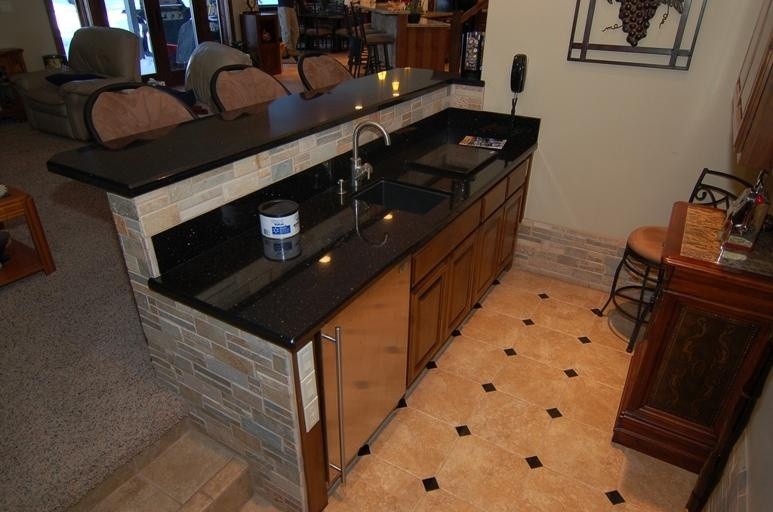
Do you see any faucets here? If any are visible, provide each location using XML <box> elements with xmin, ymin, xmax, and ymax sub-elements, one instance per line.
<box><xmin>350</xmin><ymin>119</ymin><xmax>392</xmax><ymax>184</ymax></box>
<box><xmin>353</xmin><ymin>200</ymin><xmax>390</xmax><ymax>248</ymax></box>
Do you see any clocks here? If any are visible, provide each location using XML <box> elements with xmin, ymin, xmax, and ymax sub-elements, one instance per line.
<box><xmin>247</xmin><ymin>0</ymin><xmax>256</xmax><ymax>8</ymax></box>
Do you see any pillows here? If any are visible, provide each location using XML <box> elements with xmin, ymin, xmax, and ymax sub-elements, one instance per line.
<box><xmin>45</xmin><ymin>73</ymin><xmax>108</xmax><ymax>85</ymax></box>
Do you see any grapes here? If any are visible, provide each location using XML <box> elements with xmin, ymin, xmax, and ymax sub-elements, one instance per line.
<box><xmin>619</xmin><ymin>0</ymin><xmax>660</xmax><ymax>47</ymax></box>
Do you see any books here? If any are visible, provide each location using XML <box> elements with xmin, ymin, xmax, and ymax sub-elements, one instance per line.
<box><xmin>460</xmin><ymin>134</ymin><xmax>508</xmax><ymax>150</ymax></box>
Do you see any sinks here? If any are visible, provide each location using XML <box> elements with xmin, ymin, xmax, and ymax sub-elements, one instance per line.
<box><xmin>351</xmin><ymin>179</ymin><xmax>451</xmax><ymax>216</ymax></box>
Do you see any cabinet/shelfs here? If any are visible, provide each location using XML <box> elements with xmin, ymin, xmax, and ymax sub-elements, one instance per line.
<box><xmin>314</xmin><ymin>256</ymin><xmax>410</xmax><ymax>487</ymax></box>
<box><xmin>473</xmin><ymin>157</ymin><xmax>529</xmax><ymax>307</ymax></box>
<box><xmin>409</xmin><ymin>201</ymin><xmax>483</xmax><ymax>391</ymax></box>
<box><xmin>241</xmin><ymin>11</ymin><xmax>282</xmax><ymax>75</ymax></box>
<box><xmin>611</xmin><ymin>250</ymin><xmax>773</xmax><ymax>477</ymax></box>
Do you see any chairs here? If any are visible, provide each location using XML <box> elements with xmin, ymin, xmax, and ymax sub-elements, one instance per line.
<box><xmin>600</xmin><ymin>167</ymin><xmax>755</xmax><ymax>354</ymax></box>
<box><xmin>296</xmin><ymin>0</ymin><xmax>394</xmax><ymax>77</ymax></box>
<box><xmin>210</xmin><ymin>64</ymin><xmax>293</xmax><ymax>110</ymax></box>
<box><xmin>184</xmin><ymin>42</ymin><xmax>253</xmax><ymax>119</ymax></box>
<box><xmin>10</xmin><ymin>27</ymin><xmax>142</xmax><ymax>141</ymax></box>
<box><xmin>297</xmin><ymin>53</ymin><xmax>355</xmax><ymax>90</ymax></box>
<box><xmin>83</xmin><ymin>82</ymin><xmax>200</xmax><ymax>144</ymax></box>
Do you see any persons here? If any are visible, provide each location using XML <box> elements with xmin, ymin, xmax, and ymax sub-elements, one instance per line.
<box><xmin>275</xmin><ymin>0</ymin><xmax>302</xmax><ymax>52</ymax></box>
